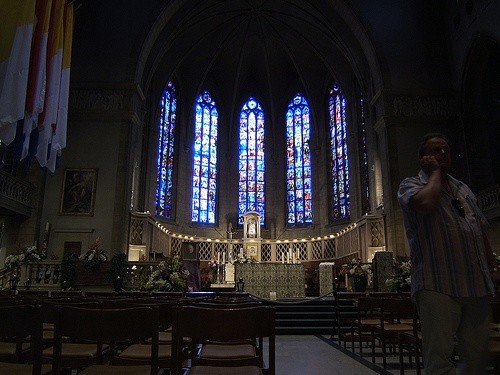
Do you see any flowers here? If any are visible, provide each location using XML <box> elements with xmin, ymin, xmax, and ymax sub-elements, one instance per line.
<box><xmin>340</xmin><ymin>258</ymin><xmax>372</xmax><ymax>284</ymax></box>
<box><xmin>385</xmin><ymin>256</ymin><xmax>414</xmax><ymax>291</ymax></box>
<box><xmin>5</xmin><ymin>241</ymin><xmax>40</xmax><ymax>267</ymax></box>
<box><xmin>79</xmin><ymin>238</ymin><xmax>108</xmax><ymax>270</ymax></box>
<box><xmin>145</xmin><ymin>260</ymin><xmax>191</xmax><ymax>291</ymax></box>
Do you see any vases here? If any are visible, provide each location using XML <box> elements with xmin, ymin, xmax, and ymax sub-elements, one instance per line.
<box><xmin>15</xmin><ymin>262</ymin><xmax>32</xmax><ymax>286</ymax></box>
<box><xmin>350</xmin><ymin>276</ymin><xmax>366</xmax><ymax>291</ymax></box>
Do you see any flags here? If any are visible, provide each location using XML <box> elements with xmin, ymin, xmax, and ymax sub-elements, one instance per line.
<box><xmin>0</xmin><ymin>0</ymin><xmax>74</xmax><ymax>175</ymax></box>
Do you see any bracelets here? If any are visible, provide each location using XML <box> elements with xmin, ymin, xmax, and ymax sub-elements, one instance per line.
<box><xmin>489</xmin><ymin>267</ymin><xmax>499</xmax><ymax>272</ymax></box>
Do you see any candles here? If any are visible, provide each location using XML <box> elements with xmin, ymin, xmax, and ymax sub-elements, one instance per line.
<box><xmin>46</xmin><ymin>222</ymin><xmax>49</xmax><ymax>232</ymax></box>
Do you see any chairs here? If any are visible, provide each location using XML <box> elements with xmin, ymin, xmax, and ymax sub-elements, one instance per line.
<box><xmin>331</xmin><ymin>292</ymin><xmax>500</xmax><ymax>375</ymax></box>
<box><xmin>0</xmin><ymin>290</ymin><xmax>278</xmax><ymax>375</ymax></box>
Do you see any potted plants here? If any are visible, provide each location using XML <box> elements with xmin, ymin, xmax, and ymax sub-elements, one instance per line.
<box><xmin>110</xmin><ymin>252</ymin><xmax>127</xmax><ymax>292</ymax></box>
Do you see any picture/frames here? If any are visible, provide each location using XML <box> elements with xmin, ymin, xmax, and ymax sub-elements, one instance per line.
<box><xmin>59</xmin><ymin>168</ymin><xmax>98</xmax><ymax>216</ymax></box>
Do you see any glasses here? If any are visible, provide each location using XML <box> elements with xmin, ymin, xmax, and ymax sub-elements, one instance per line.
<box><xmin>451</xmin><ymin>197</ymin><xmax>466</xmax><ymax>218</ymax></box>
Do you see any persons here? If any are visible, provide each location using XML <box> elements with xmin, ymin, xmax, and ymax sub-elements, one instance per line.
<box><xmin>397</xmin><ymin>132</ymin><xmax>500</xmax><ymax>375</ymax></box>
